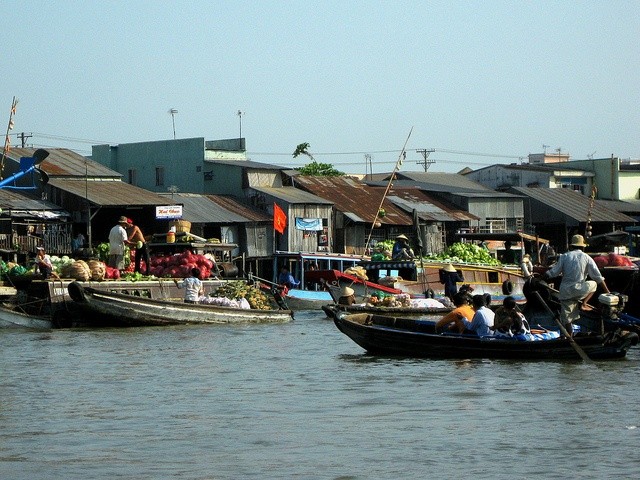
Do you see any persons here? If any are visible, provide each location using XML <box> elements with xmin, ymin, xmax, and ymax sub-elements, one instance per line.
<box><xmin>35</xmin><ymin>247</ymin><xmax>53</xmax><ymax>280</ymax></box>
<box><xmin>107</xmin><ymin>216</ymin><xmax>137</xmax><ymax>272</ymax></box>
<box><xmin>489</xmin><ymin>296</ymin><xmax>530</xmax><ymax>338</ymax></box>
<box><xmin>173</xmin><ymin>268</ymin><xmax>203</xmax><ymax>305</ymax></box>
<box><xmin>339</xmin><ymin>286</ymin><xmax>356</xmax><ymax>306</ymax></box>
<box><xmin>72</xmin><ymin>237</ymin><xmax>77</xmax><ymax>253</ymax></box>
<box><xmin>435</xmin><ymin>293</ymin><xmax>476</xmax><ymax>333</ymax></box>
<box><xmin>501</xmin><ymin>240</ymin><xmax>515</xmax><ymax>263</ymax></box>
<box><xmin>440</xmin><ymin>264</ymin><xmax>464</xmax><ymax>300</ymax></box>
<box><xmin>305</xmin><ymin>264</ymin><xmax>321</xmax><ymax>290</ymax></box>
<box><xmin>392</xmin><ymin>233</ymin><xmax>415</xmax><ymax>262</ymax></box>
<box><xmin>533</xmin><ymin>234</ymin><xmax>611</xmax><ymax>337</ymax></box>
<box><xmin>456</xmin><ymin>295</ymin><xmax>496</xmax><ymax>340</ymax></box>
<box><xmin>125</xmin><ymin>219</ymin><xmax>150</xmax><ymax>276</ymax></box>
<box><xmin>77</xmin><ymin>232</ymin><xmax>84</xmax><ymax>248</ymax></box>
<box><xmin>279</xmin><ymin>266</ymin><xmax>300</xmax><ymax>290</ymax></box>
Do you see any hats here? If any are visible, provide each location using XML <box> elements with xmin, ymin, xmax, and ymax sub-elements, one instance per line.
<box><xmin>118</xmin><ymin>216</ymin><xmax>127</xmax><ymax>223</ymax></box>
<box><xmin>443</xmin><ymin>264</ymin><xmax>457</xmax><ymax>273</ymax></box>
<box><xmin>340</xmin><ymin>287</ymin><xmax>354</xmax><ymax>296</ymax></box>
<box><xmin>37</xmin><ymin>247</ymin><xmax>44</xmax><ymax>251</ymax></box>
<box><xmin>570</xmin><ymin>235</ymin><xmax>586</xmax><ymax>247</ymax></box>
<box><xmin>396</xmin><ymin>234</ymin><xmax>408</xmax><ymax>240</ymax></box>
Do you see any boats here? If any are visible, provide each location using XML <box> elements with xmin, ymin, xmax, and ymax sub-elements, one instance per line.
<box><xmin>334</xmin><ymin>292</ymin><xmax>639</xmax><ymax>360</ymax></box>
<box><xmin>271</xmin><ymin>250</ymin><xmax>371</xmax><ymax>309</ymax></box>
<box><xmin>67</xmin><ymin>281</ymin><xmax>295</xmax><ymax>326</ymax></box>
<box><xmin>322</xmin><ymin>303</ymin><xmax>456</xmax><ymax>318</ymax></box>
<box><xmin>0</xmin><ymin>243</ymin><xmax>249</xmax><ymax>329</ymax></box>
<box><xmin>541</xmin><ymin>253</ymin><xmax>640</xmax><ymax>320</ymax></box>
<box><xmin>306</xmin><ymin>232</ymin><xmax>549</xmax><ymax>304</ymax></box>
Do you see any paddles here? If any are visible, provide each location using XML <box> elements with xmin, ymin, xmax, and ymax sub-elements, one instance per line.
<box><xmin>534</xmin><ymin>289</ymin><xmax>593</xmax><ymax>364</ymax></box>
